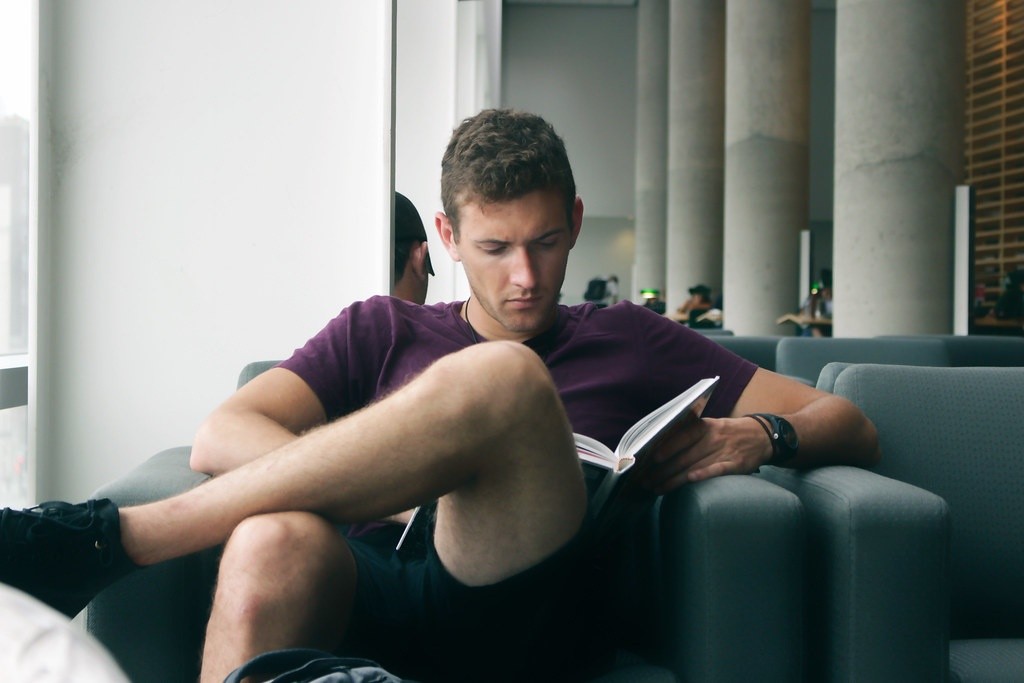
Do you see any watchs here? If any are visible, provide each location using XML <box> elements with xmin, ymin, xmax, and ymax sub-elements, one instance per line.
<box><xmin>755</xmin><ymin>413</ymin><xmax>800</xmax><ymax>461</ymax></box>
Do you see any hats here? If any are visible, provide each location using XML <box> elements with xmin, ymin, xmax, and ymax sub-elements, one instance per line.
<box><xmin>688</xmin><ymin>284</ymin><xmax>712</xmax><ymax>303</ymax></box>
<box><xmin>395</xmin><ymin>190</ymin><xmax>436</xmax><ymax>277</ymax></box>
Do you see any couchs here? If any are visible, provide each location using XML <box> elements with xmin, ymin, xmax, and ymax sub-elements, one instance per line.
<box><xmin>749</xmin><ymin>361</ymin><xmax>1024</xmax><ymax>683</ymax></box>
<box><xmin>688</xmin><ymin>328</ymin><xmax>1024</xmax><ymax>387</ymax></box>
<box><xmin>86</xmin><ymin>361</ymin><xmax>811</xmax><ymax>683</ymax></box>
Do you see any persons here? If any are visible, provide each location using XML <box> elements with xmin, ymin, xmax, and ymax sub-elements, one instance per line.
<box><xmin>803</xmin><ymin>270</ymin><xmax>831</xmax><ymax>336</ymax></box>
<box><xmin>643</xmin><ymin>293</ymin><xmax>665</xmax><ymax>315</ymax></box>
<box><xmin>1</xmin><ymin>107</ymin><xmax>880</xmax><ymax>683</ymax></box>
<box><xmin>995</xmin><ymin>269</ymin><xmax>1024</xmax><ymax>322</ymax></box>
<box><xmin>392</xmin><ymin>191</ymin><xmax>436</xmax><ymax>304</ymax></box>
<box><xmin>583</xmin><ymin>275</ymin><xmax>618</xmax><ymax>310</ymax></box>
<box><xmin>675</xmin><ymin>284</ymin><xmax>712</xmax><ymax>328</ymax></box>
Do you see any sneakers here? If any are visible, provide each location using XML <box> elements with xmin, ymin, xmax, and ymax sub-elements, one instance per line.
<box><xmin>0</xmin><ymin>497</ymin><xmax>133</xmax><ymax>618</ymax></box>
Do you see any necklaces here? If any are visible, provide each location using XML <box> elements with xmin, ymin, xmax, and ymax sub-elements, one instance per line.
<box><xmin>466</xmin><ymin>294</ymin><xmax>560</xmax><ymax>364</ymax></box>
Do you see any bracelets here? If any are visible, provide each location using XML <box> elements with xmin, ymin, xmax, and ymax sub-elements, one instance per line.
<box><xmin>742</xmin><ymin>414</ymin><xmax>776</xmax><ymax>463</ymax></box>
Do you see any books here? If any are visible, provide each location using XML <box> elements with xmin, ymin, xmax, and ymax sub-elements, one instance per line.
<box><xmin>394</xmin><ymin>376</ymin><xmax>721</xmax><ymax>552</ymax></box>
